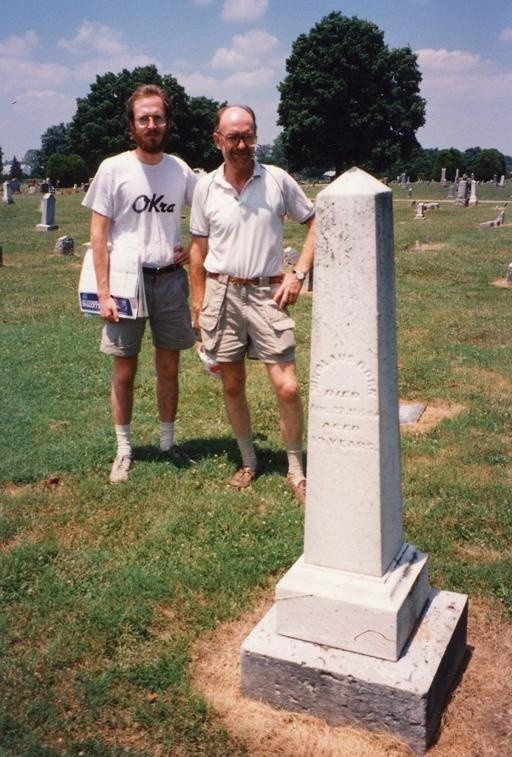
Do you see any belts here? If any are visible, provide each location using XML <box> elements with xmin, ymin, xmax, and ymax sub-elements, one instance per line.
<box><xmin>143</xmin><ymin>264</ymin><xmax>182</xmax><ymax>273</ymax></box>
<box><xmin>204</xmin><ymin>271</ymin><xmax>282</xmax><ymax>285</ymax></box>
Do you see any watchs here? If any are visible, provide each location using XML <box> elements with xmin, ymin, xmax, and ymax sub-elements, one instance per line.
<box><xmin>292</xmin><ymin>268</ymin><xmax>305</xmax><ymax>282</ymax></box>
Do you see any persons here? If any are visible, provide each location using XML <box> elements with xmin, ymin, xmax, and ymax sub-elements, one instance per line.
<box><xmin>188</xmin><ymin>105</ymin><xmax>315</xmax><ymax>503</ymax></box>
<box><xmin>82</xmin><ymin>84</ymin><xmax>200</xmax><ymax>485</ymax></box>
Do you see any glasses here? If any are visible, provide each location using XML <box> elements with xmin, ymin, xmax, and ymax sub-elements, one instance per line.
<box><xmin>135</xmin><ymin>115</ymin><xmax>166</xmax><ymax>127</ymax></box>
<box><xmin>225</xmin><ymin>134</ymin><xmax>257</xmax><ymax>146</ymax></box>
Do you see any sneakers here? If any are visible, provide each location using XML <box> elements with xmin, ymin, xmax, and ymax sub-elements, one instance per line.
<box><xmin>157</xmin><ymin>443</ymin><xmax>196</xmax><ymax>466</ymax></box>
<box><xmin>109</xmin><ymin>449</ymin><xmax>134</xmax><ymax>485</ymax></box>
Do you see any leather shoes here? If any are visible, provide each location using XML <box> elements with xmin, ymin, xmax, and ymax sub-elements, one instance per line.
<box><xmin>286</xmin><ymin>468</ymin><xmax>306</xmax><ymax>506</ymax></box>
<box><xmin>230</xmin><ymin>464</ymin><xmax>263</xmax><ymax>487</ymax></box>
